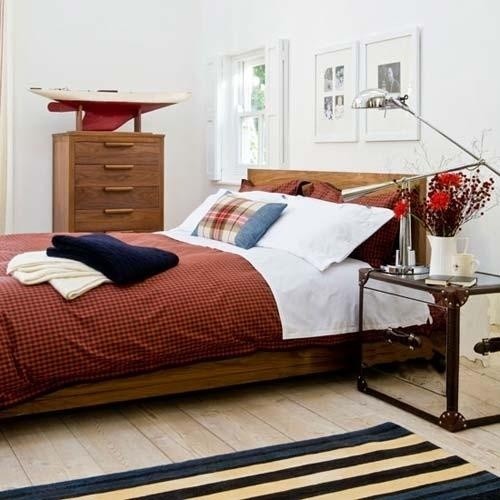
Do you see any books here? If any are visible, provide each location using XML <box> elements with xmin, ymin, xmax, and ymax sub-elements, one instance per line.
<box><xmin>425</xmin><ymin>275</ymin><xmax>477</xmax><ymax>287</ymax></box>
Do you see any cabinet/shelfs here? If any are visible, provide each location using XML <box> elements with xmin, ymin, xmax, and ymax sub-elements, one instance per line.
<box><xmin>51</xmin><ymin>132</ymin><xmax>166</xmax><ymax>235</ymax></box>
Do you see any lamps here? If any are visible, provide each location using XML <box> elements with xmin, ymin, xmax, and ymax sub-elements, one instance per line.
<box><xmin>341</xmin><ymin>89</ymin><xmax>500</xmax><ymax>276</ymax></box>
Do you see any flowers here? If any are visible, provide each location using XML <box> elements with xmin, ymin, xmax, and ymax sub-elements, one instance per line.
<box><xmin>390</xmin><ymin>168</ymin><xmax>498</xmax><ymax>237</ymax></box>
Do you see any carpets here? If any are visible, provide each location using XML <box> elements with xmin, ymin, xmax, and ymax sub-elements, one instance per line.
<box><xmin>0</xmin><ymin>420</ymin><xmax>500</xmax><ymax>499</ymax></box>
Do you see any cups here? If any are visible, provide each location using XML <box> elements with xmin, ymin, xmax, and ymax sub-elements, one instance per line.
<box><xmin>450</xmin><ymin>253</ymin><xmax>479</xmax><ymax>277</ymax></box>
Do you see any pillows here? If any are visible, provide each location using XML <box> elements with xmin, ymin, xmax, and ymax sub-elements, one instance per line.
<box><xmin>176</xmin><ymin>177</ymin><xmax>420</xmax><ymax>273</ymax></box>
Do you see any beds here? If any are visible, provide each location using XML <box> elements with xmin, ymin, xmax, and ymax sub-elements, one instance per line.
<box><xmin>0</xmin><ymin>168</ymin><xmax>429</xmax><ymax>417</ymax></box>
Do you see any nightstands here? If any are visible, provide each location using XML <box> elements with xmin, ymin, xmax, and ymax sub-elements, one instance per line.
<box><xmin>355</xmin><ymin>266</ymin><xmax>500</xmax><ymax>432</ymax></box>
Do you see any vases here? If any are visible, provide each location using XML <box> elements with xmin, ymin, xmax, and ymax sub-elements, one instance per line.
<box><xmin>426</xmin><ymin>234</ymin><xmax>469</xmax><ymax>280</ymax></box>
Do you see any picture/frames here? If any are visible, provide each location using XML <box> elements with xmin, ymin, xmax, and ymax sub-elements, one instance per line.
<box><xmin>312</xmin><ymin>27</ymin><xmax>422</xmax><ymax>144</ymax></box>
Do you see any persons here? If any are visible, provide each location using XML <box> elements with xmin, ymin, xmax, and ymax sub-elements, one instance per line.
<box><xmin>324</xmin><ymin>65</ymin><xmax>344</xmax><ymax>119</ymax></box>
<box><xmin>385</xmin><ymin>67</ymin><xmax>400</xmax><ymax>92</ymax></box>
